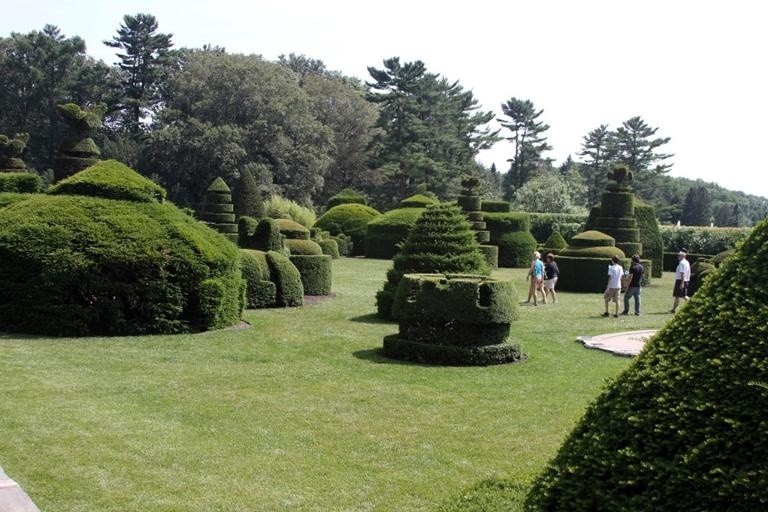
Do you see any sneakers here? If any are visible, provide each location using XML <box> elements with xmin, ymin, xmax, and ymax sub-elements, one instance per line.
<box><xmin>521</xmin><ymin>299</ymin><xmax>559</xmax><ymax>306</ymax></box>
<box><xmin>667</xmin><ymin>309</ymin><xmax>676</xmax><ymax>313</ymax></box>
<box><xmin>601</xmin><ymin>309</ymin><xmax>641</xmax><ymax>318</ymax></box>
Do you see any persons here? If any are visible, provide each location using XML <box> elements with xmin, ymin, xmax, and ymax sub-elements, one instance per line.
<box><xmin>525</xmin><ymin>250</ymin><xmax>544</xmax><ymax>306</ymax></box>
<box><xmin>529</xmin><ymin>259</ymin><xmax>548</xmax><ymax>306</ymax></box>
<box><xmin>670</xmin><ymin>250</ymin><xmax>691</xmax><ymax>314</ymax></box>
<box><xmin>542</xmin><ymin>253</ymin><xmax>561</xmax><ymax>305</ymax></box>
<box><xmin>619</xmin><ymin>254</ymin><xmax>645</xmax><ymax>316</ymax></box>
<box><xmin>601</xmin><ymin>254</ymin><xmax>625</xmax><ymax>318</ymax></box>
